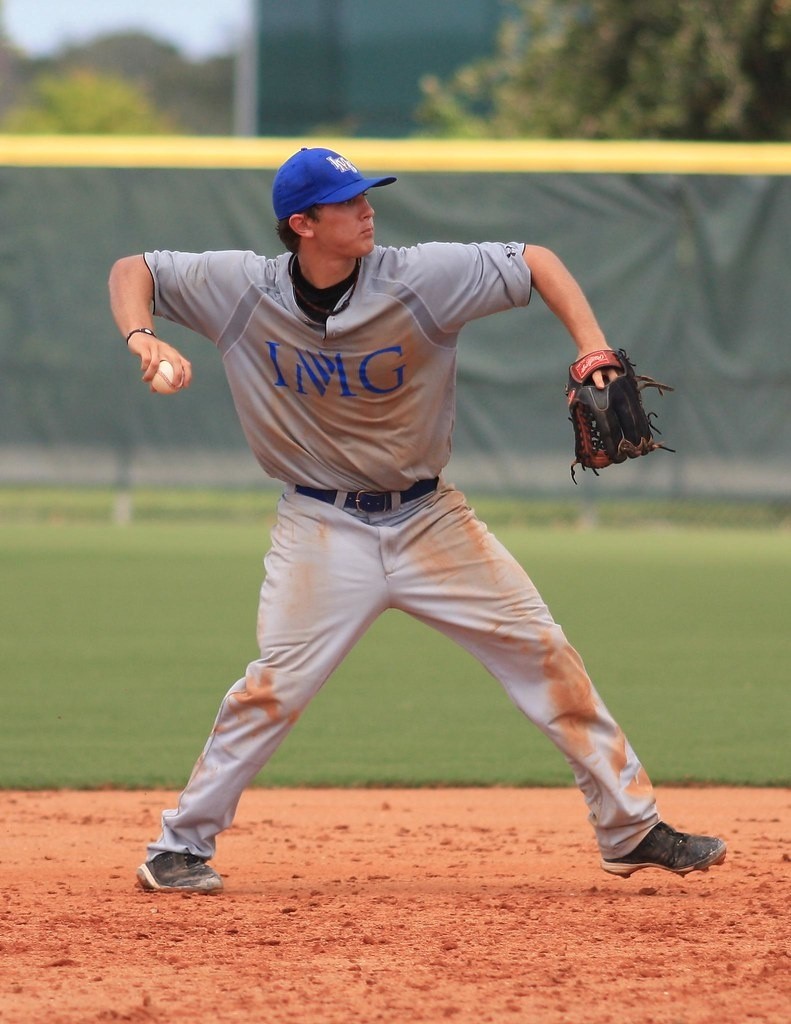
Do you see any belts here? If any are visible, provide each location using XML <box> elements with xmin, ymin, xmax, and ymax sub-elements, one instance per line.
<box><xmin>295</xmin><ymin>476</ymin><xmax>439</xmax><ymax>512</ymax></box>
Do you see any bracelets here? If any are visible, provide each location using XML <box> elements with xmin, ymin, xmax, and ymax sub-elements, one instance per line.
<box><xmin>126</xmin><ymin>328</ymin><xmax>156</xmax><ymax>345</ymax></box>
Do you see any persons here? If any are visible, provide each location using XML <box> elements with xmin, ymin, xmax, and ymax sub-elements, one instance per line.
<box><xmin>108</xmin><ymin>147</ymin><xmax>728</xmax><ymax>894</ymax></box>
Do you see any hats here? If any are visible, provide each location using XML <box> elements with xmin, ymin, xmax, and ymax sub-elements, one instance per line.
<box><xmin>271</xmin><ymin>147</ymin><xmax>397</xmax><ymax>221</ymax></box>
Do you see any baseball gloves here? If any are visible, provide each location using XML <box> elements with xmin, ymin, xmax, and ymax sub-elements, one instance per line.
<box><xmin>560</xmin><ymin>346</ymin><xmax>676</xmax><ymax>486</ymax></box>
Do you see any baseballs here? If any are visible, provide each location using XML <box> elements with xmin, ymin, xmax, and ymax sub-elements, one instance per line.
<box><xmin>150</xmin><ymin>360</ymin><xmax>186</xmax><ymax>396</ymax></box>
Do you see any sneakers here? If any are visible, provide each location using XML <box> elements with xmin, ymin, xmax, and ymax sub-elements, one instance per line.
<box><xmin>136</xmin><ymin>847</ymin><xmax>224</xmax><ymax>895</ymax></box>
<box><xmin>600</xmin><ymin>820</ymin><xmax>727</xmax><ymax>876</ymax></box>
